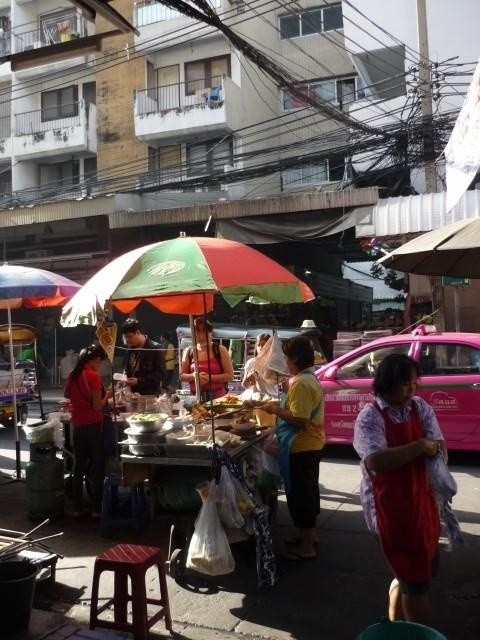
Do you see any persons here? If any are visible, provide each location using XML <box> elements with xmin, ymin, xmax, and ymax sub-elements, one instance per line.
<box><xmin>260</xmin><ymin>335</ymin><xmax>327</xmax><ymax>562</ymax></box>
<box><xmin>120</xmin><ymin>317</ymin><xmax>166</xmax><ymax>396</ymax></box>
<box><xmin>178</xmin><ymin>316</ymin><xmax>235</xmax><ymax>401</ymax></box>
<box><xmin>351</xmin><ymin>352</ymin><xmax>459</xmax><ymax>622</ymax></box>
<box><xmin>159</xmin><ymin>330</ymin><xmax>175</xmax><ymax>380</ymax></box>
<box><xmin>63</xmin><ymin>343</ymin><xmax>117</xmax><ymax>516</ymax></box>
<box><xmin>239</xmin><ymin>333</ymin><xmax>295</xmax><ymax>427</ymax></box>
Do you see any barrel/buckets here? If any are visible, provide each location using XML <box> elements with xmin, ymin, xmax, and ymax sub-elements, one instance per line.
<box><xmin>0</xmin><ymin>558</ymin><xmax>40</xmax><ymax>634</ymax></box>
<box><xmin>332</xmin><ymin>328</ymin><xmax>392</xmax><ymax>357</ymax></box>
<box><xmin>358</xmin><ymin>617</ymin><xmax>446</xmax><ymax>640</ymax></box>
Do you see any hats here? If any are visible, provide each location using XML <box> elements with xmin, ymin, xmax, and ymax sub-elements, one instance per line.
<box><xmin>421</xmin><ymin>316</ymin><xmax>433</xmax><ymax>324</ymax></box>
<box><xmin>300</xmin><ymin>320</ymin><xmax>317</xmax><ymax>328</ymax></box>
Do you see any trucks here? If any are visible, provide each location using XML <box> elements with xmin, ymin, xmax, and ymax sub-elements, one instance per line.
<box><xmin>0</xmin><ymin>359</ymin><xmax>37</xmax><ymax>427</ymax></box>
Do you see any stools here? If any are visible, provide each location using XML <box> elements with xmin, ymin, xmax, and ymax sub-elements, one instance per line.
<box><xmin>89</xmin><ymin>542</ymin><xmax>173</xmax><ymax>638</ymax></box>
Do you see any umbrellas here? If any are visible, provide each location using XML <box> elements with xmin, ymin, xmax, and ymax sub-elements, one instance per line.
<box><xmin>375</xmin><ymin>217</ymin><xmax>480</xmax><ymax>279</ymax></box>
<box><xmin>60</xmin><ymin>230</ymin><xmax>316</xmax><ymax>403</ymax></box>
<box><xmin>0</xmin><ymin>260</ymin><xmax>84</xmax><ymax>478</ymax></box>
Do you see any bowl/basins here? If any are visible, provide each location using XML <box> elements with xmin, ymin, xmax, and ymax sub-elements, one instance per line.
<box><xmin>233</xmin><ymin>420</ymin><xmax>257</xmax><ymax>432</ymax></box>
<box><xmin>124</xmin><ymin>414</ymin><xmax>170</xmax><ymax>456</ymax></box>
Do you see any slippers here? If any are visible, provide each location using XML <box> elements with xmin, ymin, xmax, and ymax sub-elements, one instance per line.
<box><xmin>279</xmin><ymin>536</ymin><xmax>320</xmax><ymax>562</ymax></box>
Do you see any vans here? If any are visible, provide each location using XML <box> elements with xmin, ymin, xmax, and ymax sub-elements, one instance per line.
<box><xmin>176</xmin><ymin>320</ymin><xmax>329</xmax><ymax>413</ymax></box>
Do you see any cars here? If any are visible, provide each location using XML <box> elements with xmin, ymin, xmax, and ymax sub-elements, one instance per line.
<box><xmin>314</xmin><ymin>324</ymin><xmax>480</xmax><ymax>457</ymax></box>
